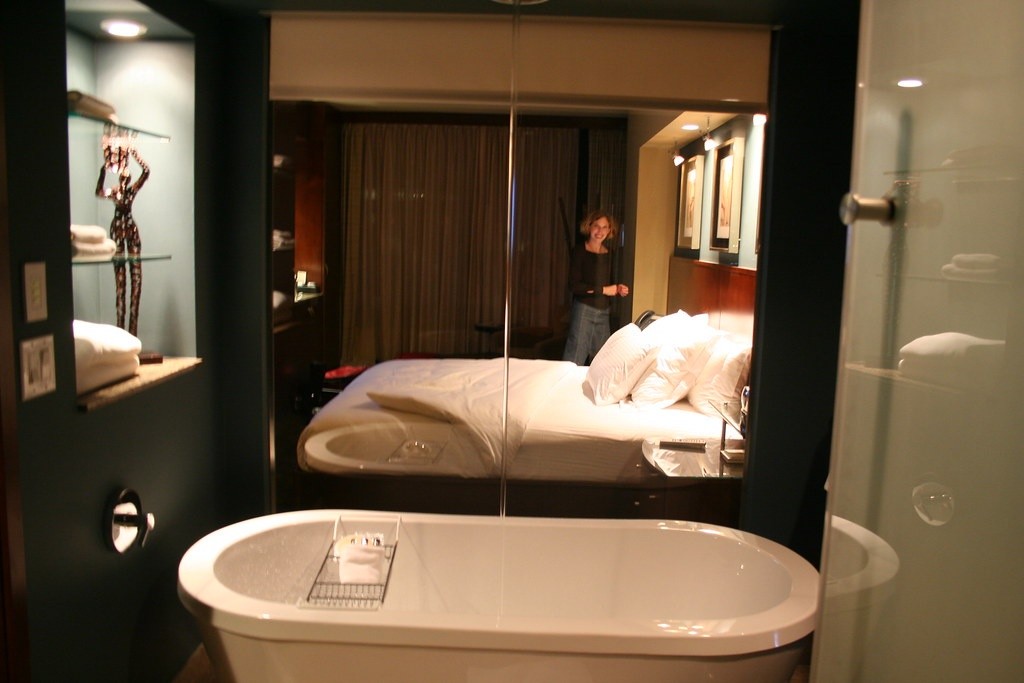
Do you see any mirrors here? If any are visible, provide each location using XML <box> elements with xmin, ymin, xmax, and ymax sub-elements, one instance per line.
<box><xmin>258</xmin><ymin>11</ymin><xmax>774</xmax><ymax>527</ymax></box>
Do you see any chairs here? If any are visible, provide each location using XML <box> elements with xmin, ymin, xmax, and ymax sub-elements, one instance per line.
<box><xmin>491</xmin><ymin>325</ymin><xmax>553</xmax><ymax>360</ymax></box>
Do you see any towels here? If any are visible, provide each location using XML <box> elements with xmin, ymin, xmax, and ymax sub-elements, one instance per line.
<box><xmin>75</xmin><ymin>317</ymin><xmax>144</xmax><ymax>392</ymax></box>
<box><xmin>72</xmin><ymin>224</ymin><xmax>118</xmax><ymax>264</ymax></box>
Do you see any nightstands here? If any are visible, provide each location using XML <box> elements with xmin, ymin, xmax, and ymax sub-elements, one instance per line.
<box><xmin>636</xmin><ymin>437</ymin><xmax>744</xmax><ymax>530</ymax></box>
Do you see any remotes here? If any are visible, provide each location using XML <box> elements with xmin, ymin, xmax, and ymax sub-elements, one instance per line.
<box><xmin>659</xmin><ymin>437</ymin><xmax>706</xmax><ymax>447</ymax></box>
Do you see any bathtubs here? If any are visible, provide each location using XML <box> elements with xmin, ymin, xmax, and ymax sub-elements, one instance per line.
<box><xmin>179</xmin><ymin>509</ymin><xmax>820</xmax><ymax>683</ymax></box>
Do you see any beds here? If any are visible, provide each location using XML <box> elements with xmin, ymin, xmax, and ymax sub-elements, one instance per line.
<box><xmin>299</xmin><ymin>356</ymin><xmax>747</xmax><ymax>490</ymax></box>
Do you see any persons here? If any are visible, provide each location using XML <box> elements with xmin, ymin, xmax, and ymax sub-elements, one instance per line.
<box><xmin>95</xmin><ymin>146</ymin><xmax>150</xmax><ymax>336</ymax></box>
<box><xmin>564</xmin><ymin>209</ymin><xmax>628</xmax><ymax>366</ymax></box>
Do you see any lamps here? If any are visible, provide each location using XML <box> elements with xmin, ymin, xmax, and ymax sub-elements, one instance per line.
<box><xmin>669</xmin><ymin>140</ymin><xmax>684</xmax><ymax>167</ymax></box>
<box><xmin>698</xmin><ymin>125</ymin><xmax>718</xmax><ymax>152</ymax></box>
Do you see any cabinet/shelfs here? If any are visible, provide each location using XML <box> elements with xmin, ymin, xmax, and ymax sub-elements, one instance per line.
<box><xmin>270</xmin><ymin>140</ymin><xmax>327</xmax><ymax>334</ymax></box>
<box><xmin>62</xmin><ymin>0</ymin><xmax>203</xmax><ymax>415</ymax></box>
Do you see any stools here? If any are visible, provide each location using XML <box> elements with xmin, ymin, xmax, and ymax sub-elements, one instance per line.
<box><xmin>322</xmin><ymin>365</ymin><xmax>367</xmax><ymax>405</ymax></box>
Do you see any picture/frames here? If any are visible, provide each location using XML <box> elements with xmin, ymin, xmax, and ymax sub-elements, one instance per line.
<box><xmin>673</xmin><ymin>155</ymin><xmax>705</xmax><ymax>260</ymax></box>
<box><xmin>709</xmin><ymin>137</ymin><xmax>744</xmax><ymax>254</ymax></box>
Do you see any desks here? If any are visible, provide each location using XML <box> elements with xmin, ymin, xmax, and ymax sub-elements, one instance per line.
<box><xmin>476</xmin><ymin>320</ymin><xmax>505</xmax><ymax>357</ymax></box>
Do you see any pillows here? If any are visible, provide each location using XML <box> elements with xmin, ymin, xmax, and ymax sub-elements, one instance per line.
<box><xmin>586</xmin><ymin>308</ymin><xmax>752</xmax><ymax>418</ymax></box>
